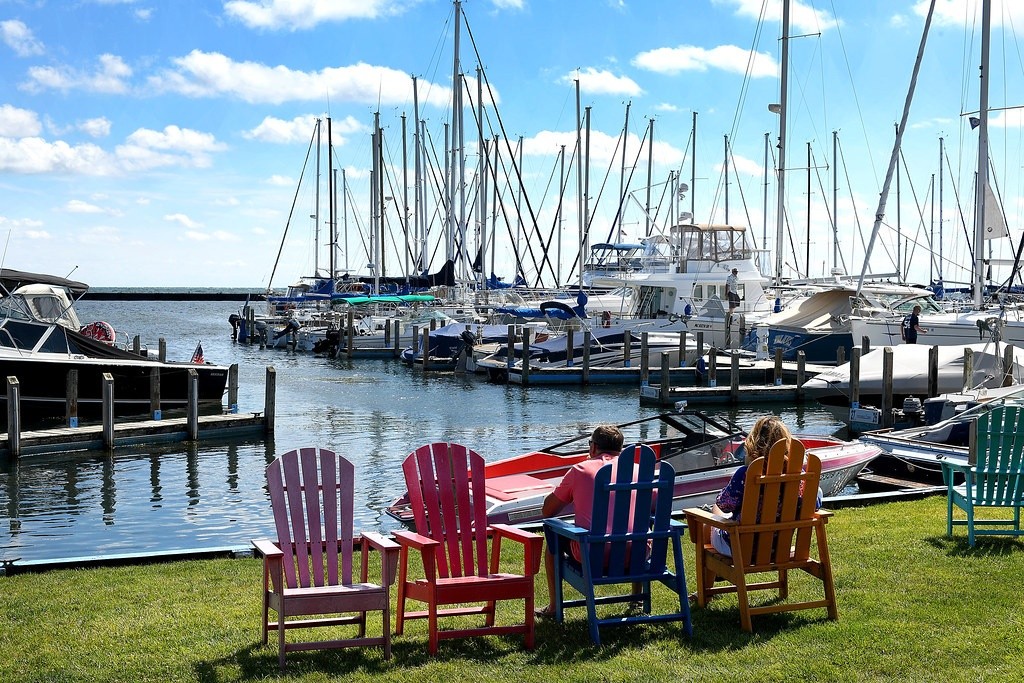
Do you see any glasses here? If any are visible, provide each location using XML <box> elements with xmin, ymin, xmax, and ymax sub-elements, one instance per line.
<box><xmin>588</xmin><ymin>440</ymin><xmax>592</xmax><ymax>446</ymax></box>
<box><xmin>918</xmin><ymin>309</ymin><xmax>921</xmax><ymax>312</ymax></box>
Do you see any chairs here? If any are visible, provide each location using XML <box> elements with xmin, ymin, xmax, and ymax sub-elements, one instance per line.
<box><xmin>542</xmin><ymin>444</ymin><xmax>693</xmax><ymax>646</ymax></box>
<box><xmin>940</xmin><ymin>405</ymin><xmax>1024</xmax><ymax>547</ymax></box>
<box><xmin>389</xmin><ymin>442</ymin><xmax>545</xmax><ymax>658</ymax></box>
<box><xmin>251</xmin><ymin>448</ymin><xmax>402</xmax><ymax>671</ymax></box>
<box><xmin>680</xmin><ymin>437</ymin><xmax>839</xmax><ymax>633</ymax></box>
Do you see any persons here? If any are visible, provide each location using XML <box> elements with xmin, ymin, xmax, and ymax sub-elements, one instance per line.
<box><xmin>688</xmin><ymin>415</ymin><xmax>822</xmax><ymax>602</ymax></box>
<box><xmin>901</xmin><ymin>306</ymin><xmax>927</xmax><ymax>344</ymax></box>
<box><xmin>725</xmin><ymin>268</ymin><xmax>740</xmax><ymax>313</ymax></box>
<box><xmin>534</xmin><ymin>425</ymin><xmax>658</xmax><ymax>617</ymax></box>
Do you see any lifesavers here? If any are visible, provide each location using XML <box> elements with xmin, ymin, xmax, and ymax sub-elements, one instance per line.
<box><xmin>602</xmin><ymin>311</ymin><xmax>611</xmax><ymax>328</ymax></box>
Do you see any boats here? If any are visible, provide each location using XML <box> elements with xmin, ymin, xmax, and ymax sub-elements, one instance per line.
<box><xmin>383</xmin><ymin>409</ymin><xmax>883</xmax><ymax>537</ymax></box>
<box><xmin>0</xmin><ymin>227</ymin><xmax>228</xmax><ymax>418</ymax></box>
<box><xmin>858</xmin><ymin>378</ymin><xmax>1024</xmax><ymax>486</ymax></box>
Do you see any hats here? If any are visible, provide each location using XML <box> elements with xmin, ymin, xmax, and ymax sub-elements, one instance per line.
<box><xmin>732</xmin><ymin>268</ymin><xmax>739</xmax><ymax>272</ymax></box>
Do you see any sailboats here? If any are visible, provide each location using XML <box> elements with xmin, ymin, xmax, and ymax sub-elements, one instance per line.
<box><xmin>231</xmin><ymin>0</ymin><xmax>1024</xmax><ymax>385</ymax></box>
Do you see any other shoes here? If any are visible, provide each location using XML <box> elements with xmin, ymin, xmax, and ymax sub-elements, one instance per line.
<box><xmin>534</xmin><ymin>605</ymin><xmax>565</xmax><ymax>623</ymax></box>
<box><xmin>631</xmin><ymin>601</ymin><xmax>643</xmax><ymax>612</ymax></box>
<box><xmin>689</xmin><ymin>592</ymin><xmax>714</xmax><ymax>605</ymax></box>
